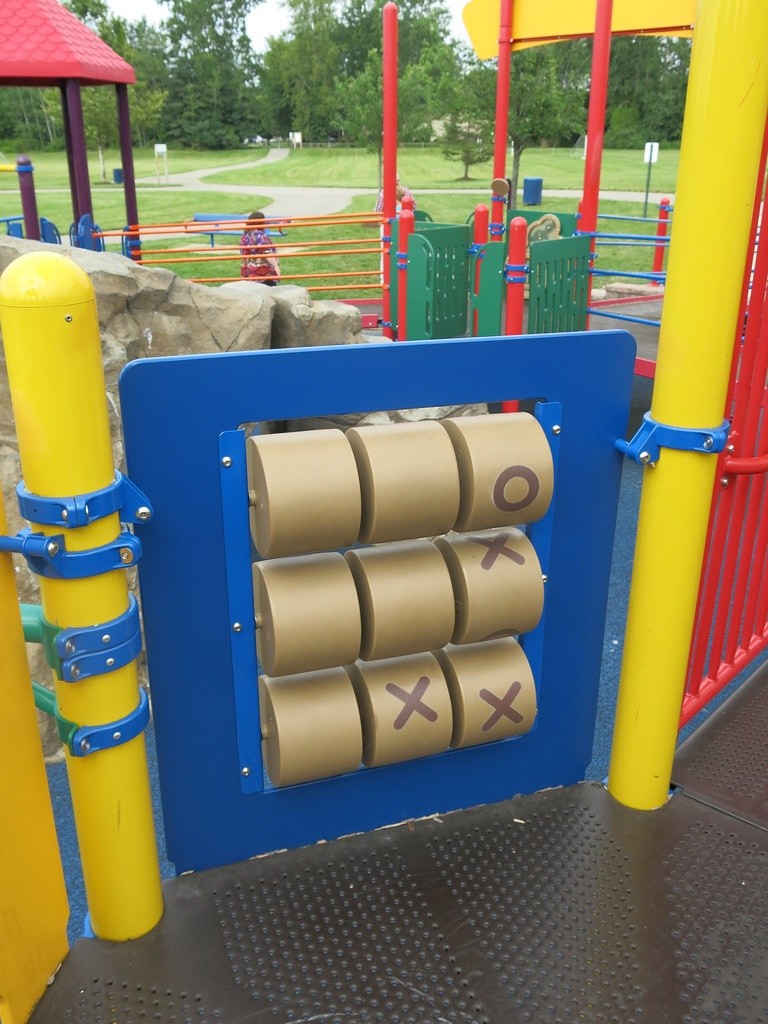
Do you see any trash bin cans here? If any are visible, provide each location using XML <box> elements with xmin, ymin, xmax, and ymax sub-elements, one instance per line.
<box><xmin>522</xmin><ymin>177</ymin><xmax>543</xmax><ymax>205</ymax></box>
<box><xmin>113</xmin><ymin>168</ymin><xmax>123</xmax><ymax>184</ymax></box>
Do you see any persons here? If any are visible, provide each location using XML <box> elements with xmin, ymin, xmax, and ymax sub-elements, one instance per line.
<box><xmin>375</xmin><ymin>174</ymin><xmax>415</xmax><ymax>284</ymax></box>
<box><xmin>240</xmin><ymin>212</ymin><xmax>280</xmax><ymax>286</ymax></box>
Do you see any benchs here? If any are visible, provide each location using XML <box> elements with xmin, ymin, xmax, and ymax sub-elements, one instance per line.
<box><xmin>186</xmin><ymin>214</ymin><xmax>289</xmax><ymax>247</ymax></box>
